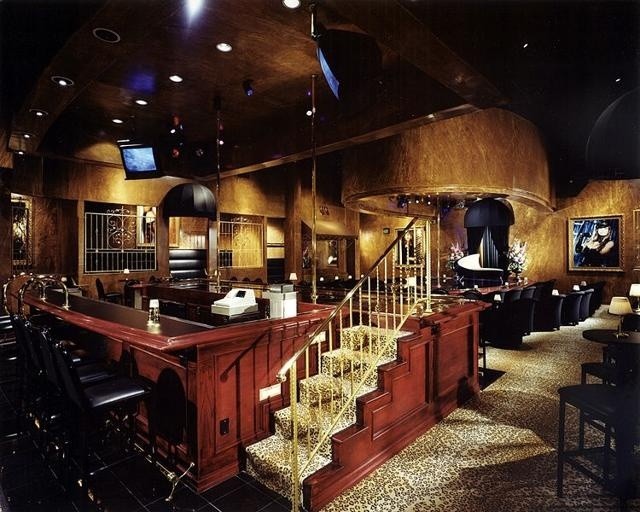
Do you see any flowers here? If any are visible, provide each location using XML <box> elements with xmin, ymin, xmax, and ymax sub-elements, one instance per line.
<box><xmin>445</xmin><ymin>240</ymin><xmax>465</xmax><ymax>269</ymax></box>
<box><xmin>504</xmin><ymin>237</ymin><xmax>528</xmax><ymax>272</ymax></box>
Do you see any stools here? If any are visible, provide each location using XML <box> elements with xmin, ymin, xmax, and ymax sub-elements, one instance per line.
<box><xmin>553</xmin><ymin>283</ymin><xmax>640</xmax><ymax>512</ymax></box>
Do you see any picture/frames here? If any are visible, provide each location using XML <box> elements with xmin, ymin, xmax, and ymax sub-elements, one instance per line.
<box><xmin>393</xmin><ymin>226</ymin><xmax>426</xmax><ymax>269</ymax></box>
<box><xmin>565</xmin><ymin>212</ymin><xmax>626</xmax><ymax>274</ymax></box>
<box><xmin>9</xmin><ymin>192</ymin><xmax>35</xmax><ymax>265</ymax></box>
<box><xmin>631</xmin><ymin>208</ymin><xmax>640</xmax><ymax>270</ymax></box>
<box><xmin>135</xmin><ymin>206</ymin><xmax>181</xmax><ymax>248</ymax></box>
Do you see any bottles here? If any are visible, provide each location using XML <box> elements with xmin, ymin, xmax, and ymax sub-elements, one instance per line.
<box><xmin>146</xmin><ymin>298</ymin><xmax>162</xmax><ymax>328</ymax></box>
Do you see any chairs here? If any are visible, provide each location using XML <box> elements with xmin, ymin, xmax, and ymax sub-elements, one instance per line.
<box><xmin>1</xmin><ymin>297</ymin><xmax>163</xmax><ymax>512</ymax></box>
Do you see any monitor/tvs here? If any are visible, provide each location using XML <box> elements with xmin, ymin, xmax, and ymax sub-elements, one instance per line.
<box><xmin>119</xmin><ymin>142</ymin><xmax>164</xmax><ymax>179</ymax></box>
